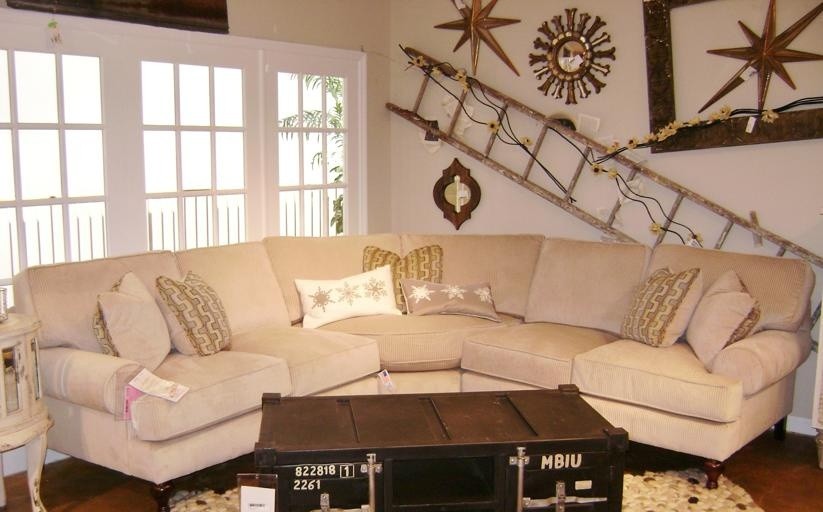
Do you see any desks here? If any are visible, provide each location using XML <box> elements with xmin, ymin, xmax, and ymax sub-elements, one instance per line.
<box><xmin>0</xmin><ymin>316</ymin><xmax>54</xmax><ymax>511</ymax></box>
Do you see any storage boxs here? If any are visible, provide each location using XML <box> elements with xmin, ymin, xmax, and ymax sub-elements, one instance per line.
<box><xmin>256</xmin><ymin>381</ymin><xmax>632</xmax><ymax>510</ymax></box>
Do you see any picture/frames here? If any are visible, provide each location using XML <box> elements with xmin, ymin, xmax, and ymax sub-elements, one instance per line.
<box><xmin>641</xmin><ymin>1</ymin><xmax>823</xmax><ymax>155</ymax></box>
<box><xmin>2</xmin><ymin>2</ymin><xmax>229</xmax><ymax>36</ymax></box>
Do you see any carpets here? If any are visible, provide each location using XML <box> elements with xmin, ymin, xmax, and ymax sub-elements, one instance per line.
<box><xmin>166</xmin><ymin>464</ymin><xmax>769</xmax><ymax>512</ymax></box>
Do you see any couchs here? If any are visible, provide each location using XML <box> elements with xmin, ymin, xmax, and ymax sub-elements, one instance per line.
<box><xmin>12</xmin><ymin>230</ymin><xmax>814</xmax><ymax>512</ymax></box>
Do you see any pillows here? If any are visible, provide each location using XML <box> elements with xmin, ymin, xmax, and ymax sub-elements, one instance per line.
<box><xmin>292</xmin><ymin>264</ymin><xmax>404</xmax><ymax>329</ymax></box>
<box><xmin>401</xmin><ymin>276</ymin><xmax>500</xmax><ymax>324</ymax></box>
<box><xmin>684</xmin><ymin>265</ymin><xmax>762</xmax><ymax>374</ymax></box>
<box><xmin>358</xmin><ymin>240</ymin><xmax>444</xmax><ymax>319</ymax></box>
<box><xmin>92</xmin><ymin>270</ymin><xmax>174</xmax><ymax>374</ymax></box>
<box><xmin>153</xmin><ymin>266</ymin><xmax>231</xmax><ymax>359</ymax></box>
<box><xmin>616</xmin><ymin>265</ymin><xmax>703</xmax><ymax>347</ymax></box>
